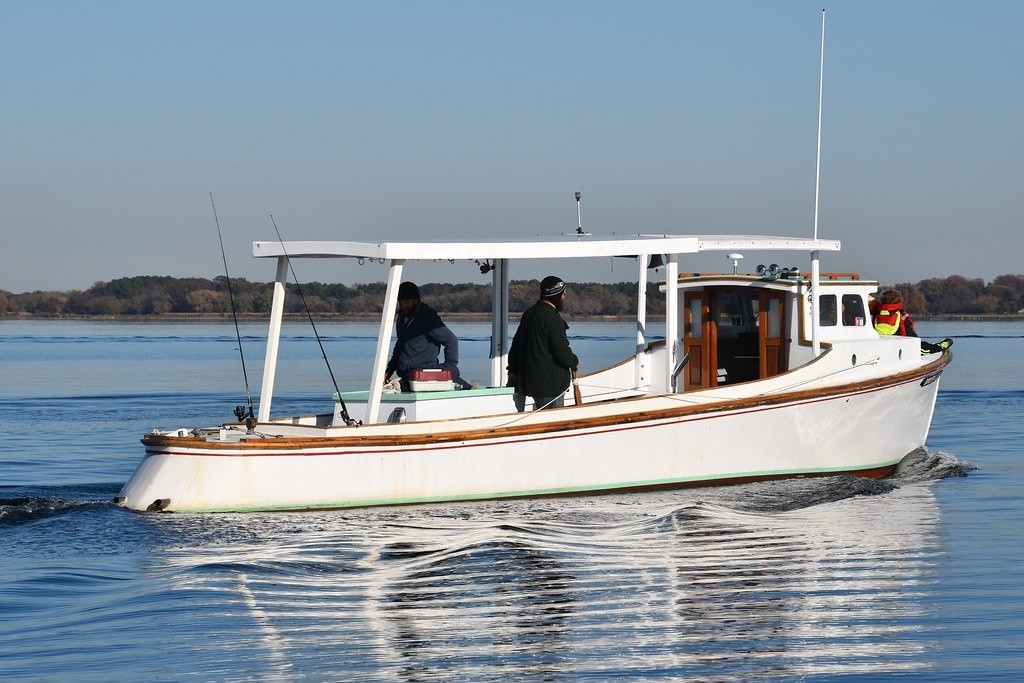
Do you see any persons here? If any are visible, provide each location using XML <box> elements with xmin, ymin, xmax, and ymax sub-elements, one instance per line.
<box><xmin>505</xmin><ymin>275</ymin><xmax>579</xmax><ymax>412</ymax></box>
<box><xmin>873</xmin><ymin>290</ymin><xmax>955</xmax><ymax>358</ymax></box>
<box><xmin>383</xmin><ymin>280</ymin><xmax>460</xmax><ymax>392</ymax></box>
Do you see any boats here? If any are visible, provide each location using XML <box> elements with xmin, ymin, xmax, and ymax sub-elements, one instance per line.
<box><xmin>115</xmin><ymin>4</ymin><xmax>963</xmax><ymax>516</ymax></box>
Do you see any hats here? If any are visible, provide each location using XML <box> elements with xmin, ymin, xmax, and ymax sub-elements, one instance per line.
<box><xmin>541</xmin><ymin>274</ymin><xmax>567</xmax><ymax>298</ymax></box>
<box><xmin>398</xmin><ymin>281</ymin><xmax>421</xmax><ymax>300</ymax></box>
